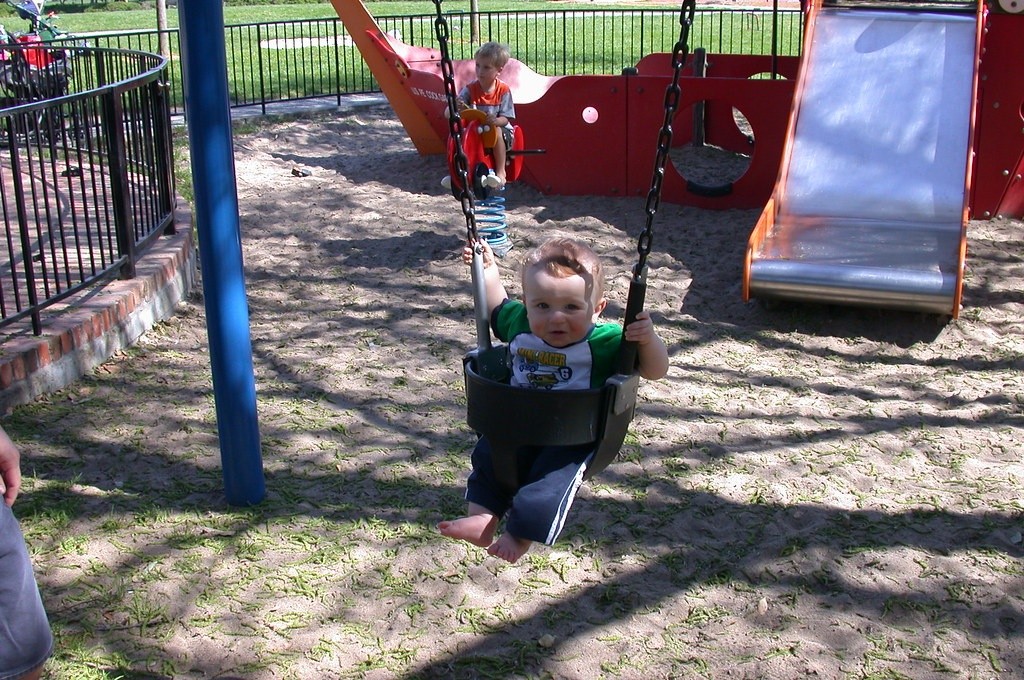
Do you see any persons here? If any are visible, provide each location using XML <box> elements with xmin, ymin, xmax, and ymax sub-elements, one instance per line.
<box><xmin>441</xmin><ymin>41</ymin><xmax>515</xmax><ymax>189</ymax></box>
<box><xmin>438</xmin><ymin>236</ymin><xmax>668</xmax><ymax>564</ymax></box>
<box><xmin>0</xmin><ymin>424</ymin><xmax>55</xmax><ymax>680</ymax></box>
<box><xmin>0</xmin><ymin>23</ymin><xmax>8</xmax><ymax>35</ymax></box>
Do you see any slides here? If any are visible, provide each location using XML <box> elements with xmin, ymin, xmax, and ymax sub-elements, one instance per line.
<box><xmin>739</xmin><ymin>0</ymin><xmax>988</xmax><ymax>321</ymax></box>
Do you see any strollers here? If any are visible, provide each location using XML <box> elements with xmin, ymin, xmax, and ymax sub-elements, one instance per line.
<box><xmin>0</xmin><ymin>0</ymin><xmax>85</xmax><ymax>145</ymax></box>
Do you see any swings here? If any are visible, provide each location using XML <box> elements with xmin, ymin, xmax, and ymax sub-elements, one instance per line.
<box><xmin>427</xmin><ymin>0</ymin><xmax>702</xmax><ymax>504</ymax></box>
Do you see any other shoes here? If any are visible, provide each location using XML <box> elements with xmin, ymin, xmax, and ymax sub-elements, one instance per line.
<box><xmin>441</xmin><ymin>175</ymin><xmax>451</xmax><ymax>190</ymax></box>
<box><xmin>486</xmin><ymin>174</ymin><xmax>505</xmax><ymax>190</ymax></box>
